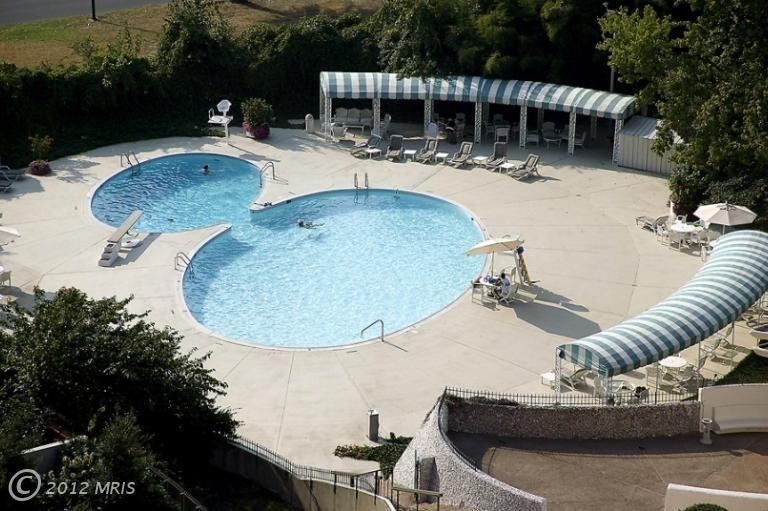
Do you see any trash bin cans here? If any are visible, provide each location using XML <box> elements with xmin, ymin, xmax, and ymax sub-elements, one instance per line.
<box><xmin>304</xmin><ymin>112</ymin><xmax>314</xmax><ymax>133</ymax></box>
<box><xmin>367</xmin><ymin>410</ymin><xmax>379</xmax><ymax>440</ymax></box>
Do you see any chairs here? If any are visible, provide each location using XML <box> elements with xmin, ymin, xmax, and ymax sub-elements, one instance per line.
<box><xmin>330</xmin><ymin>108</ymin><xmax>584</xmax><ymax>182</ymax></box>
<box><xmin>538</xmin><ymin>353</ymin><xmax>708</xmax><ymax>404</ymax></box>
<box><xmin>471</xmin><ymin>265</ymin><xmax>518</xmax><ymax>307</ymax></box>
<box><xmin>635</xmin><ymin>213</ymin><xmax>711</xmax><ymax>251</ymax></box>
<box><xmin>699</xmin><ymin>325</ymin><xmax>733</xmax><ymax>357</ymax></box>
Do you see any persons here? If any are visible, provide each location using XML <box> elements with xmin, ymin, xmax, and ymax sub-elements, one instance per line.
<box><xmin>491</xmin><ymin>273</ymin><xmax>510</xmax><ymax>302</ymax></box>
<box><xmin>634</xmin><ymin>386</ymin><xmax>647</xmax><ymax>403</ymax></box>
<box><xmin>199</xmin><ymin>164</ymin><xmax>213</xmax><ymax>174</ymax></box>
<box><xmin>606</xmin><ymin>397</ymin><xmax>614</xmax><ymax>407</ymax></box>
<box><xmin>295</xmin><ymin>219</ymin><xmax>325</xmax><ymax>230</ymax></box>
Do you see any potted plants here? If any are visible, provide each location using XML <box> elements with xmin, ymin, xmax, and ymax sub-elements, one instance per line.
<box><xmin>241</xmin><ymin>97</ymin><xmax>272</xmax><ymax>139</ymax></box>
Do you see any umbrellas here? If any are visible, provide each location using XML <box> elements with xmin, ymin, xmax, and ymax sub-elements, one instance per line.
<box><xmin>693</xmin><ymin>200</ymin><xmax>757</xmax><ymax>234</ymax></box>
<box><xmin>0</xmin><ymin>224</ymin><xmax>21</xmax><ymax>246</ymax></box>
<box><xmin>463</xmin><ymin>237</ymin><xmax>526</xmax><ymax>277</ymax></box>
